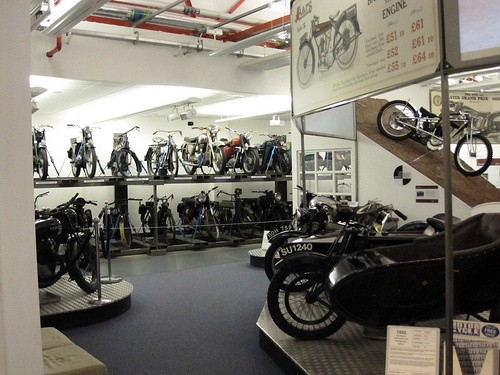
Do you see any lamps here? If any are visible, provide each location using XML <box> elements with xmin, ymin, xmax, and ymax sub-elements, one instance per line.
<box><xmin>167</xmin><ymin>104</ymin><xmax>196</xmax><ymax>121</ymax></box>
<box><xmin>270</xmin><ymin>115</ymin><xmax>284</xmax><ymax>127</ymax></box>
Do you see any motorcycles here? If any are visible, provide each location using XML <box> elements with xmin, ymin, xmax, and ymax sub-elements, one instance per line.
<box><xmin>295</xmin><ymin>3</ymin><xmax>363</xmax><ymax>88</ymax></box>
<box><xmin>176</xmin><ymin>185</ymin><xmax>294</xmax><ymax>249</ymax></box>
<box><xmin>262</xmin><ymin>184</ymin><xmax>500</xmax><ymax>342</ymax></box>
<box><xmin>138</xmin><ymin>194</ymin><xmax>176</xmax><ymax>241</ymax></box>
<box><xmin>34</xmin><ymin>192</ymin><xmax>101</xmax><ymax>294</ymax></box>
<box><xmin>375</xmin><ymin>99</ymin><xmax>493</xmax><ymax>177</ymax></box>
<box><xmin>67</xmin><ymin>123</ymin><xmax>101</xmax><ymax>178</ymax></box>
<box><xmin>98</xmin><ymin>198</ymin><xmax>137</xmax><ymax>250</ymax></box>
<box><xmin>31</xmin><ymin>125</ymin><xmax>54</xmax><ymax>180</ymax></box>
<box><xmin>143</xmin><ymin>125</ymin><xmax>293</xmax><ymax>178</ymax></box>
<box><xmin>108</xmin><ymin>125</ymin><xmax>142</xmax><ymax>179</ymax></box>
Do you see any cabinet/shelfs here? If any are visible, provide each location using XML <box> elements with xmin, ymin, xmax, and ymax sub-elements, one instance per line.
<box><xmin>34</xmin><ymin>173</ymin><xmax>292</xmax><ymax>255</ymax></box>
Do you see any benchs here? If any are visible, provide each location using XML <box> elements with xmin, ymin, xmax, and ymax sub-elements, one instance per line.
<box><xmin>40</xmin><ymin>327</ymin><xmax>108</xmax><ymax>375</ymax></box>
